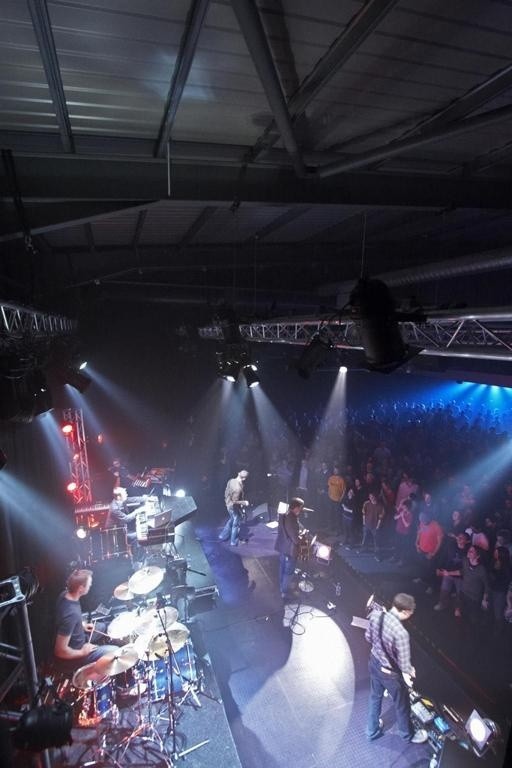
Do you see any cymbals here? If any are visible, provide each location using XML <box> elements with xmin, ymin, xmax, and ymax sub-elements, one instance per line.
<box><xmin>129</xmin><ymin>566</ymin><xmax>167</xmax><ymax>594</ymax></box>
<box><xmin>114</xmin><ymin>581</ymin><xmax>134</xmax><ymax>601</ymax></box>
<box><xmin>96</xmin><ymin>642</ymin><xmax>141</xmax><ymax>675</ymax></box>
<box><xmin>133</xmin><ymin>620</ymin><xmax>189</xmax><ymax>663</ymax></box>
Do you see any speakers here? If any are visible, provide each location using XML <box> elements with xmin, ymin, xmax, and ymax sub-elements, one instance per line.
<box><xmin>253</xmin><ymin>502</ymin><xmax>271</xmax><ymax>522</ymax></box>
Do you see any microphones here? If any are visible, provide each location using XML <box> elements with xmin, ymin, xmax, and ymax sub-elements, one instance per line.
<box><xmin>302</xmin><ymin>508</ymin><xmax>314</xmax><ymax>512</ymax></box>
<box><xmin>267</xmin><ymin>473</ymin><xmax>272</xmax><ymax>477</ymax></box>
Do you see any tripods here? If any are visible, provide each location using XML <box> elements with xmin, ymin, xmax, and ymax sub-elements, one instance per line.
<box><xmin>82</xmin><ymin>598</ymin><xmax>223</xmax><ymax>768</ymax></box>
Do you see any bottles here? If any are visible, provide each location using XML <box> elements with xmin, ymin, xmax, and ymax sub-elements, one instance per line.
<box><xmin>139</xmin><ymin>511</ymin><xmax>147</xmax><ymax>533</ymax></box>
<box><xmin>335</xmin><ymin>582</ymin><xmax>342</xmax><ymax>596</ymax></box>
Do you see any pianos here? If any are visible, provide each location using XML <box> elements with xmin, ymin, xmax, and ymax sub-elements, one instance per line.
<box><xmin>135</xmin><ymin>493</ymin><xmax>175</xmax><ymax>545</ymax></box>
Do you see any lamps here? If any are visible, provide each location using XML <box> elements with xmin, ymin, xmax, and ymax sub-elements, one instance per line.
<box><xmin>0</xmin><ymin>328</ymin><xmax>91</xmax><ymax>469</ymax></box>
<box><xmin>213</xmin><ymin>269</ymin><xmax>423</xmax><ymax>389</ymax></box>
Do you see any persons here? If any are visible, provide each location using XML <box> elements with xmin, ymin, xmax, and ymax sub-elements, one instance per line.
<box><xmin>105</xmin><ymin>487</ymin><xmax>145</xmax><ymax>544</ymax></box>
<box><xmin>108</xmin><ymin>458</ymin><xmax>138</xmax><ymax>487</ymax></box>
<box><xmin>274</xmin><ymin>496</ymin><xmax>306</xmax><ymax>600</ymax></box>
<box><xmin>219</xmin><ymin>397</ymin><xmax>510</xmax><ymax>625</ymax></box>
<box><xmin>364</xmin><ymin>593</ymin><xmax>427</xmax><ymax>742</ymax></box>
<box><xmin>53</xmin><ymin>568</ymin><xmax>120</xmax><ymax>675</ymax></box>
<box><xmin>220</xmin><ymin>470</ymin><xmax>250</xmax><ymax>547</ymax></box>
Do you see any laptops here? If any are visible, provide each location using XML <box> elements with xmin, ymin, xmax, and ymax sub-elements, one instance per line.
<box><xmin>149</xmin><ymin>510</ymin><xmax>172</xmax><ymax>528</ymax></box>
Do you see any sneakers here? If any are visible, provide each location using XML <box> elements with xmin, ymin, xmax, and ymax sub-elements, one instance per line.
<box><xmin>230</xmin><ymin>539</ymin><xmax>246</xmax><ymax>546</ymax></box>
<box><xmin>367</xmin><ymin>718</ymin><xmax>383</xmax><ymax>741</ymax></box>
<box><xmin>281</xmin><ymin>590</ymin><xmax>302</xmax><ymax>599</ymax></box>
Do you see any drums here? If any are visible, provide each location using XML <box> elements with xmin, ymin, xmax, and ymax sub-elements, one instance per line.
<box><xmin>108</xmin><ymin>611</ymin><xmax>139</xmax><ymax>645</ymax></box>
<box><xmin>72</xmin><ymin>663</ymin><xmax>116</xmax><ymax>718</ymax></box>
<box><xmin>149</xmin><ymin>640</ymin><xmax>198</xmax><ymax>706</ymax></box>
